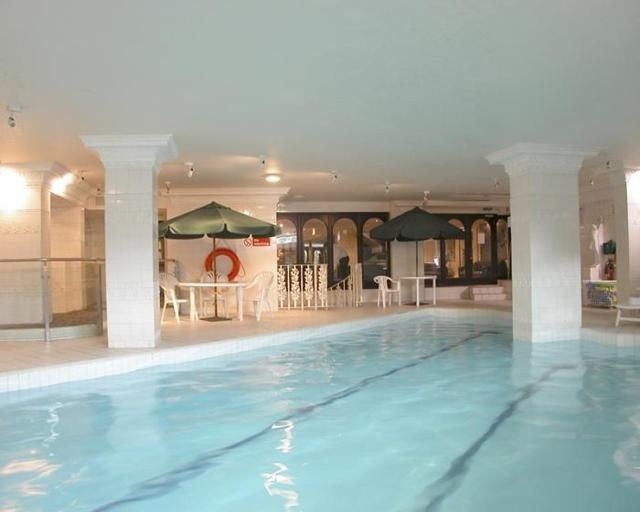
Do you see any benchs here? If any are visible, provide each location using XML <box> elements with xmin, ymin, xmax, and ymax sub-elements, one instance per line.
<box><xmin>469</xmin><ymin>286</ymin><xmax>507</xmax><ymax>302</ymax></box>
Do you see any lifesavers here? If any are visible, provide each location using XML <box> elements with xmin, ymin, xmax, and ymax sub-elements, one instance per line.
<box><xmin>204</xmin><ymin>249</ymin><xmax>241</xmax><ymax>280</ymax></box>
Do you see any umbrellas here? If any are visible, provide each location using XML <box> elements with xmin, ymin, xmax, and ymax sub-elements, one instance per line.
<box><xmin>157</xmin><ymin>199</ymin><xmax>279</xmax><ymax>316</ymax></box>
<box><xmin>369</xmin><ymin>205</ymin><xmax>466</xmax><ymax>277</ymax></box>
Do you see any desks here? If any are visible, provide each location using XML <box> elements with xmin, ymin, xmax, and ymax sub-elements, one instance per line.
<box><xmin>583</xmin><ymin>279</ymin><xmax>617</xmax><ymax>309</ymax></box>
<box><xmin>613</xmin><ymin>303</ymin><xmax>640</xmax><ymax>327</ymax></box>
<box><xmin>400</xmin><ymin>274</ymin><xmax>437</xmax><ymax>309</ymax></box>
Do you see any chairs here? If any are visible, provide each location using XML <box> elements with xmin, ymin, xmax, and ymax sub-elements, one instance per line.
<box><xmin>373</xmin><ymin>275</ymin><xmax>401</xmax><ymax>309</ymax></box>
<box><xmin>156</xmin><ymin>270</ymin><xmax>275</xmax><ymax>325</ymax></box>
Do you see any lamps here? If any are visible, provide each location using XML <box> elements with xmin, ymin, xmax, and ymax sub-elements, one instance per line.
<box><xmin>260</xmin><ymin>169</ymin><xmax>284</xmax><ymax>183</ymax></box>
